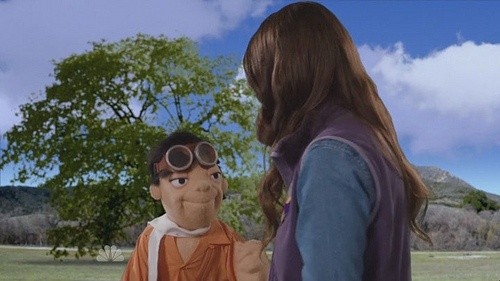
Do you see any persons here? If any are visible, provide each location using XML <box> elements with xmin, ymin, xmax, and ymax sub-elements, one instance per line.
<box><xmin>121</xmin><ymin>132</ymin><xmax>270</xmax><ymax>281</ymax></box>
<box><xmin>242</xmin><ymin>1</ymin><xmax>435</xmax><ymax>281</ymax></box>
